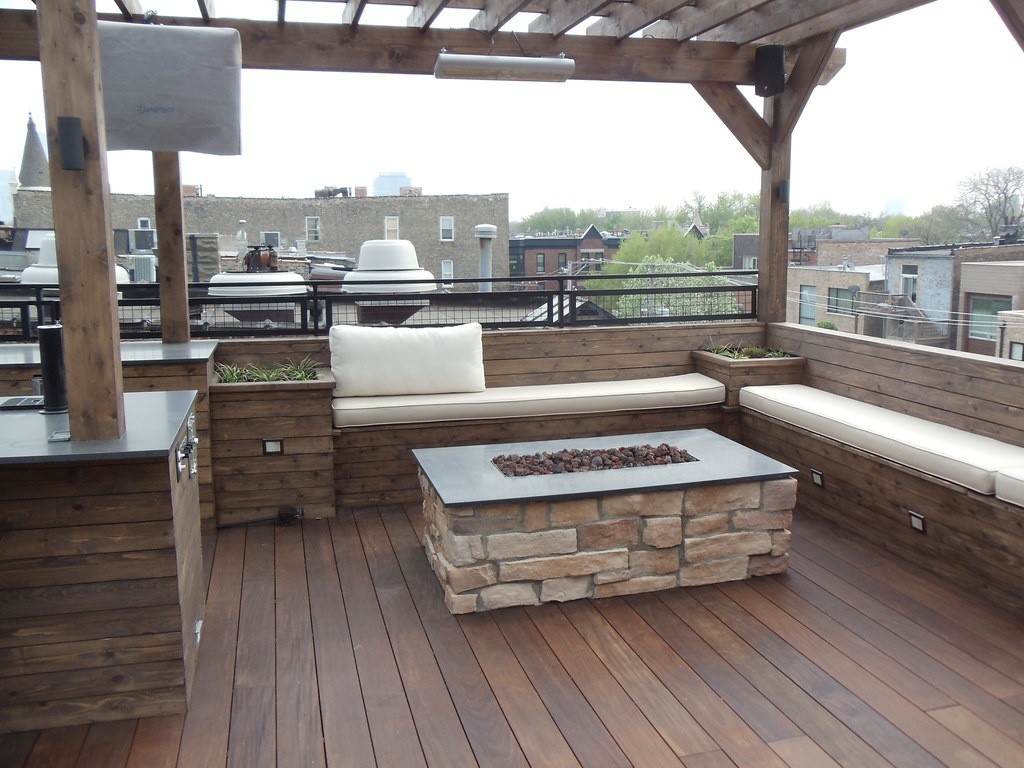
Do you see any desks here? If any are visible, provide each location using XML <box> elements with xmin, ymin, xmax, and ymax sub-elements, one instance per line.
<box><xmin>412</xmin><ymin>426</ymin><xmax>798</xmax><ymax>616</ymax></box>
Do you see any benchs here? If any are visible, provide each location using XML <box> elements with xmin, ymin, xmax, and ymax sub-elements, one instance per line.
<box><xmin>740</xmin><ymin>383</ymin><xmax>1024</xmax><ymax>620</ymax></box>
<box><xmin>329</xmin><ymin>371</ymin><xmax>727</xmax><ymax>508</ymax></box>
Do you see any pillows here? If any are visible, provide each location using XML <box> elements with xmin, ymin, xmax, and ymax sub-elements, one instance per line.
<box><xmin>328</xmin><ymin>322</ymin><xmax>486</xmax><ymax>399</ymax></box>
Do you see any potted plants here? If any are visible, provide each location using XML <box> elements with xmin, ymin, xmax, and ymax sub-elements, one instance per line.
<box><xmin>691</xmin><ymin>333</ymin><xmax>808</xmax><ymax>406</ymax></box>
<box><xmin>208</xmin><ymin>352</ymin><xmax>340</xmax><ymax>526</ymax></box>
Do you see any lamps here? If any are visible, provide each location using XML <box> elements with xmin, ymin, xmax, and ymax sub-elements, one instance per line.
<box><xmin>908</xmin><ymin>509</ymin><xmax>926</xmax><ymax>535</ymax></box>
<box><xmin>809</xmin><ymin>468</ymin><xmax>825</xmax><ymax>489</ymax></box>
<box><xmin>262</xmin><ymin>438</ymin><xmax>284</xmax><ymax>455</ymax></box>
